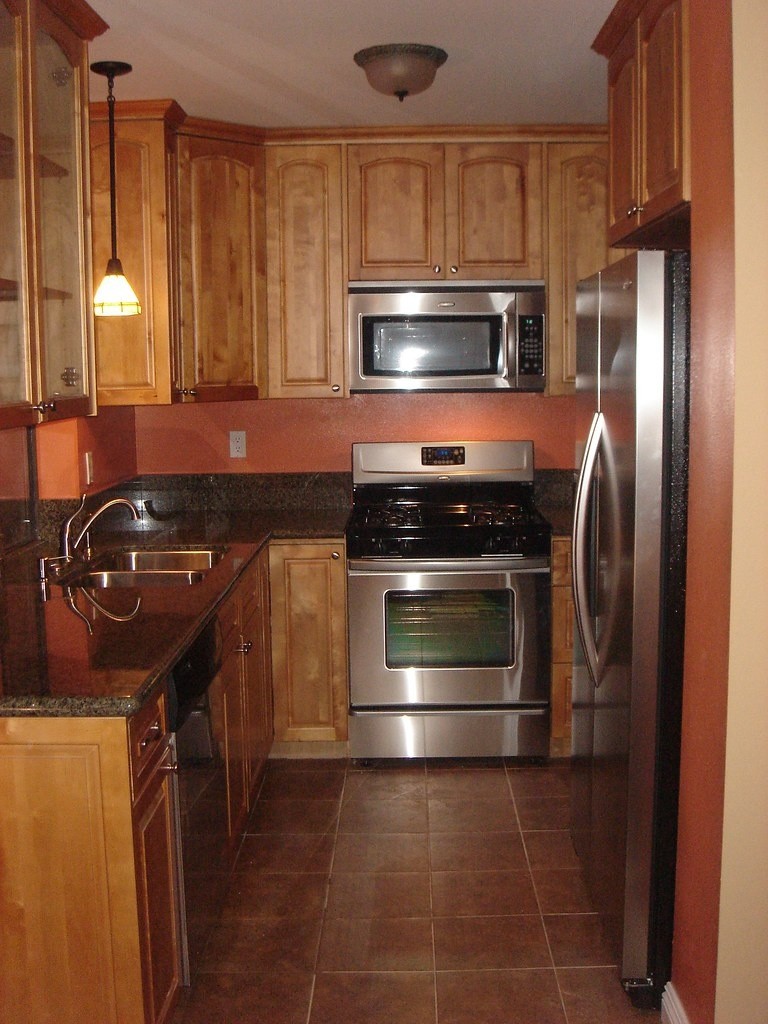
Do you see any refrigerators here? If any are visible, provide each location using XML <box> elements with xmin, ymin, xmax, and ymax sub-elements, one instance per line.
<box><xmin>563</xmin><ymin>243</ymin><xmax>683</xmax><ymax>1008</ymax></box>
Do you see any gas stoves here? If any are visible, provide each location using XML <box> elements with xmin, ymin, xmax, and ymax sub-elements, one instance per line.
<box><xmin>346</xmin><ymin>439</ymin><xmax>553</xmax><ymax>564</ymax></box>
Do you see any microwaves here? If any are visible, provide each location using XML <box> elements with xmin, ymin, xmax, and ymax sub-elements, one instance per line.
<box><xmin>344</xmin><ymin>284</ymin><xmax>552</xmax><ymax>393</ymax></box>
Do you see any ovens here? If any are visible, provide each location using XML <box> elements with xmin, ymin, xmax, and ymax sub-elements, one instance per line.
<box><xmin>340</xmin><ymin>557</ymin><xmax>551</xmax><ymax>760</ymax></box>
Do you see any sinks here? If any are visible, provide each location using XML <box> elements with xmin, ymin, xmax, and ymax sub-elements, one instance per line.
<box><xmin>90</xmin><ymin>548</ymin><xmax>221</xmax><ymax>571</ymax></box>
<box><xmin>61</xmin><ymin>569</ymin><xmax>209</xmax><ymax>585</ymax></box>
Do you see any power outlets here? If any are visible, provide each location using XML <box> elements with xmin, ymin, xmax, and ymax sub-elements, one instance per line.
<box><xmin>229</xmin><ymin>431</ymin><xmax>246</xmax><ymax>457</ymax></box>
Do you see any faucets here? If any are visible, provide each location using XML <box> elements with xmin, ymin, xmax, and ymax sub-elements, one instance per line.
<box><xmin>58</xmin><ymin>492</ymin><xmax>141</xmax><ymax>559</ymax></box>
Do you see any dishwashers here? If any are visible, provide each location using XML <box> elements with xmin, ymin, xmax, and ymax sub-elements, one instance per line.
<box><xmin>158</xmin><ymin>621</ymin><xmax>240</xmax><ymax>989</ymax></box>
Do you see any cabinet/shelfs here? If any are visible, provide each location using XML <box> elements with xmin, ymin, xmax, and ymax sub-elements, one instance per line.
<box><xmin>266</xmin><ymin>127</ymin><xmax>346</xmax><ymax>398</ymax></box>
<box><xmin>203</xmin><ymin>545</ymin><xmax>274</xmax><ymax>874</ymax></box>
<box><xmin>267</xmin><ymin>537</ymin><xmax>349</xmax><ymax>759</ymax></box>
<box><xmin>547</xmin><ymin>124</ymin><xmax>639</xmax><ymax>396</ymax></box>
<box><xmin>88</xmin><ymin>99</ymin><xmax>266</xmax><ymax>405</ymax></box>
<box><xmin>550</xmin><ymin>535</ymin><xmax>572</xmax><ymax>763</ymax></box>
<box><xmin>1</xmin><ymin>0</ymin><xmax>111</xmax><ymax>427</ymax></box>
<box><xmin>0</xmin><ymin>676</ymin><xmax>186</xmax><ymax>1024</ymax></box>
<box><xmin>590</xmin><ymin>0</ymin><xmax>691</xmax><ymax>253</ymax></box>
<box><xmin>343</xmin><ymin>124</ymin><xmax>548</xmax><ymax>280</ymax></box>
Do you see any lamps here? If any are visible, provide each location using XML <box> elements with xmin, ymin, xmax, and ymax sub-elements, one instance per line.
<box><xmin>90</xmin><ymin>61</ymin><xmax>139</xmax><ymax>316</ymax></box>
<box><xmin>352</xmin><ymin>43</ymin><xmax>448</xmax><ymax>103</ymax></box>
